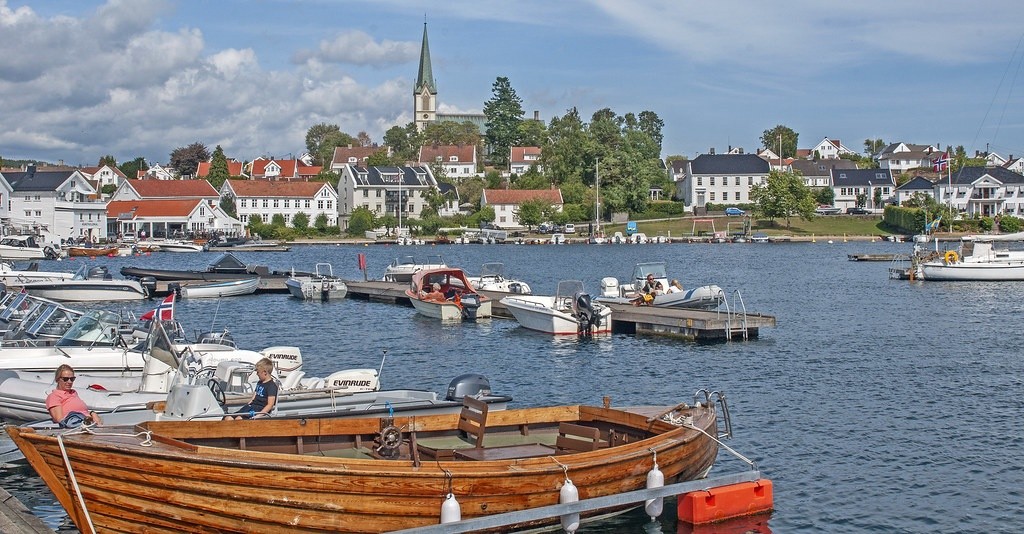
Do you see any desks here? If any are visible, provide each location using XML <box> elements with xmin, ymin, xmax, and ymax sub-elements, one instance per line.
<box><xmin>452</xmin><ymin>444</ymin><xmax>555</xmax><ymax>462</ymax></box>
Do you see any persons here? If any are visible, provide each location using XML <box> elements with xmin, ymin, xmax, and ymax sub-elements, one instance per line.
<box><xmin>627</xmin><ymin>274</ymin><xmax>665</xmax><ymax>303</ymax></box>
<box><xmin>141</xmin><ymin>229</ymin><xmax>146</xmax><ymax>240</ymax></box>
<box><xmin>46</xmin><ymin>365</ymin><xmax>103</xmax><ymax>425</ymax></box>
<box><xmin>85</xmin><ymin>240</ymin><xmax>91</xmax><ymax>247</ymax></box>
<box><xmin>694</xmin><ymin>206</ymin><xmax>698</xmax><ymax>216</ymax></box>
<box><xmin>223</xmin><ymin>358</ymin><xmax>277</xmax><ymax>420</ymax></box>
<box><xmin>667</xmin><ymin>280</ymin><xmax>684</xmax><ymax>294</ymax></box>
<box><xmin>419</xmin><ymin>283</ymin><xmax>444</xmax><ymax>300</ymax></box>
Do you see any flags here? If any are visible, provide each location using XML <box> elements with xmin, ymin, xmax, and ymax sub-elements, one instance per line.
<box><xmin>932</xmin><ymin>153</ymin><xmax>947</xmax><ymax>172</ymax></box>
<box><xmin>925</xmin><ymin>216</ymin><xmax>942</xmax><ymax>230</ymax></box>
<box><xmin>140</xmin><ymin>294</ymin><xmax>173</xmax><ymax>321</ymax></box>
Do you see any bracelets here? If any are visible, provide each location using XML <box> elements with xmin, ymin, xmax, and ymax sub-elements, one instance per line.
<box><xmin>90</xmin><ymin>411</ymin><xmax>94</xmax><ymax>416</ymax></box>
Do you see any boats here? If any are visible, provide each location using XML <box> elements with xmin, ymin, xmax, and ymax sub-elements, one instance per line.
<box><xmin>710</xmin><ymin>231</ymin><xmax>770</xmax><ymax>244</ymax></box>
<box><xmin>499</xmin><ymin>278</ymin><xmax>614</xmax><ymax>335</ymax></box>
<box><xmin>381</xmin><ymin>254</ymin><xmax>446</xmax><ymax>283</ymax></box>
<box><xmin>846</xmin><ymin>253</ymin><xmax>913</xmax><ymax>262</ymax></box>
<box><xmin>0</xmin><ymin>391</ymin><xmax>737</xmax><ymax>534</ymax></box>
<box><xmin>1</xmin><ymin>222</ymin><xmax>514</xmax><ymax>442</ymax></box>
<box><xmin>283</xmin><ymin>262</ymin><xmax>348</xmax><ymax>300</ymax></box>
<box><xmin>403</xmin><ymin>267</ymin><xmax>494</xmax><ymax>320</ymax></box>
<box><xmin>442</xmin><ymin>260</ymin><xmax>532</xmax><ymax>295</ymax></box>
<box><xmin>584</xmin><ymin>261</ymin><xmax>727</xmax><ymax>312</ymax></box>
<box><xmin>587</xmin><ymin>158</ymin><xmax>667</xmax><ymax>244</ymax></box>
<box><xmin>889</xmin><ymin>211</ymin><xmax>1024</xmax><ymax>282</ymax></box>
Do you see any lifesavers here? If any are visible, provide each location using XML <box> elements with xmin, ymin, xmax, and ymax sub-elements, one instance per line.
<box><xmin>945</xmin><ymin>251</ymin><xmax>958</xmax><ymax>263</ymax></box>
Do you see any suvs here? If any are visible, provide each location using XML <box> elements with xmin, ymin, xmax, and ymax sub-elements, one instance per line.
<box><xmin>725</xmin><ymin>207</ymin><xmax>746</xmax><ymax>216</ymax></box>
<box><xmin>847</xmin><ymin>207</ymin><xmax>873</xmax><ymax>215</ymax></box>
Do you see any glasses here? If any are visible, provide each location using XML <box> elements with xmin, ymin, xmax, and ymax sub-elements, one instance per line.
<box><xmin>649</xmin><ymin>278</ymin><xmax>654</xmax><ymax>280</ymax></box>
<box><xmin>60</xmin><ymin>377</ymin><xmax>76</xmax><ymax>382</ymax></box>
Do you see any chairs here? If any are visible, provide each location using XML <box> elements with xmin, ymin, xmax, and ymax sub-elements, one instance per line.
<box><xmin>416</xmin><ymin>393</ymin><xmax>489</xmax><ymax>461</ymax></box>
<box><xmin>262</xmin><ymin>378</ymin><xmax>280</xmax><ymax>417</ymax></box>
<box><xmin>553</xmin><ymin>422</ymin><xmax>601</xmax><ymax>455</ymax></box>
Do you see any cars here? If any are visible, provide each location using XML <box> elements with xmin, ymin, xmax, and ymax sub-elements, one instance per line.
<box><xmin>537</xmin><ymin>224</ymin><xmax>575</xmax><ymax>235</ymax></box>
